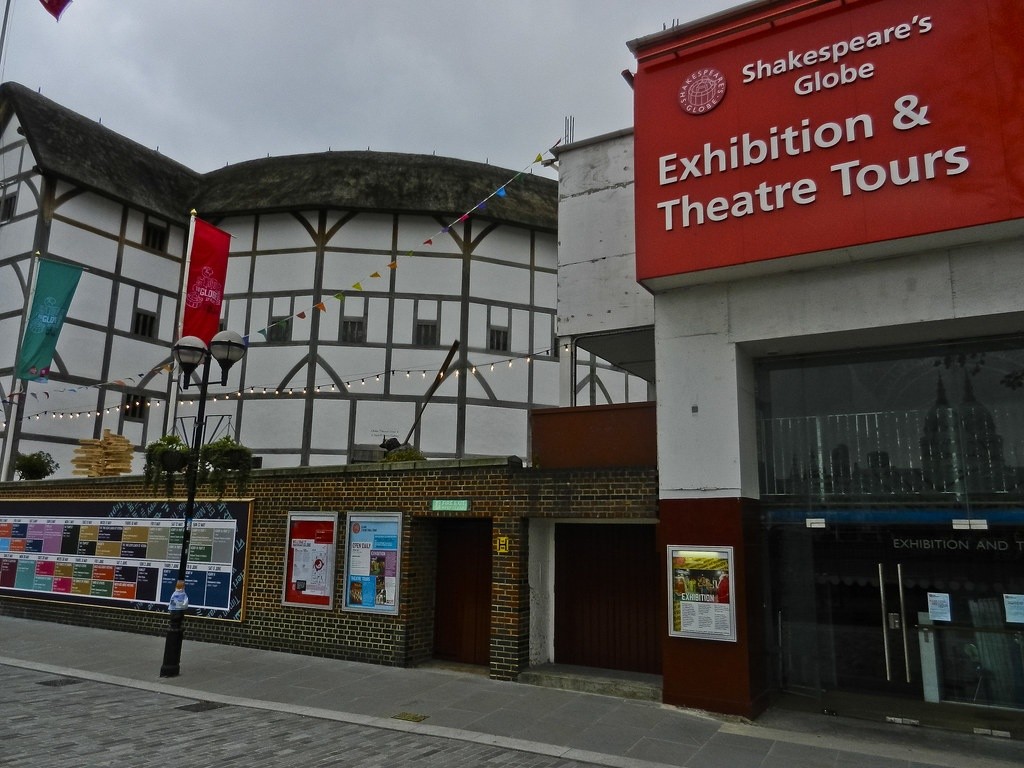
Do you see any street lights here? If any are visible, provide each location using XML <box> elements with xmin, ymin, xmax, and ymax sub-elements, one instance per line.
<box><xmin>157</xmin><ymin>328</ymin><xmax>248</xmax><ymax>677</ymax></box>
